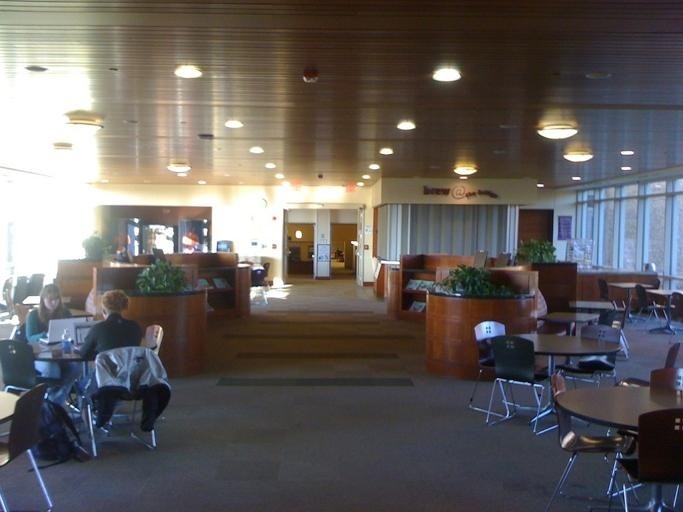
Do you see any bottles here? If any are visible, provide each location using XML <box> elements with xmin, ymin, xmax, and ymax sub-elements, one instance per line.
<box><xmin>61</xmin><ymin>327</ymin><xmax>71</xmax><ymax>353</ymax></box>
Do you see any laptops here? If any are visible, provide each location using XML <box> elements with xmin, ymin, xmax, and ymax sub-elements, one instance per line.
<box><xmin>73</xmin><ymin>320</ymin><xmax>101</xmax><ymax>351</ymax></box>
<box><xmin>39</xmin><ymin>317</ymin><xmax>87</xmax><ymax>345</ymax></box>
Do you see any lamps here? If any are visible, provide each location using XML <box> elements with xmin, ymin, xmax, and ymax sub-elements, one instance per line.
<box><xmin>164</xmin><ymin>161</ymin><xmax>192</xmax><ymax>172</ymax></box>
<box><xmin>451</xmin><ymin>113</ymin><xmax>596</xmax><ymax>178</ymax></box>
<box><xmin>58</xmin><ymin>115</ymin><xmax>104</xmax><ymax>140</ymax></box>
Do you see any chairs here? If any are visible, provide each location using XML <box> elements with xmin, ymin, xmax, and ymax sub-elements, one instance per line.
<box><xmin>467</xmin><ymin>319</ymin><xmax>622</xmax><ymax>437</ymax></box>
<box><xmin>537</xmin><ymin>278</ymin><xmax>682</xmax><ymax>362</ymax></box>
<box><xmin>546</xmin><ymin>343</ymin><xmax>682</xmax><ymax>512</ymax></box>
<box><xmin>0</xmin><ymin>272</ymin><xmax>169</xmax><ymax>512</ymax></box>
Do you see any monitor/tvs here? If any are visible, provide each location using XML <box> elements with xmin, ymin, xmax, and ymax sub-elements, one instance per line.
<box><xmin>473</xmin><ymin>249</ymin><xmax>488</xmax><ymax>267</ymax></box>
<box><xmin>495</xmin><ymin>253</ymin><xmax>511</xmax><ymax>267</ymax></box>
<box><xmin>152</xmin><ymin>248</ymin><xmax>166</xmax><ymax>263</ymax></box>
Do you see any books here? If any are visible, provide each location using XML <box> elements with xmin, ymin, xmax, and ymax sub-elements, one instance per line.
<box><xmin>198</xmin><ymin>278</ymin><xmax>227</xmax><ymax>289</ymax></box>
<box><xmin>405</xmin><ymin>278</ymin><xmax>436</xmax><ymax>312</ymax></box>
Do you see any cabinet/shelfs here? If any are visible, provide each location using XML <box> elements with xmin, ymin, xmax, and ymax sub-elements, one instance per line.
<box><xmin>384</xmin><ymin>251</ymin><xmax>536</xmax><ymax>383</ymax></box>
<box><xmin>50</xmin><ymin>251</ymin><xmax>271</xmax><ymax>379</ymax></box>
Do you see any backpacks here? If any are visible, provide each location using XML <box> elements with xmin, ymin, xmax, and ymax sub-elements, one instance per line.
<box><xmin>1</xmin><ymin>339</ymin><xmax>37</xmax><ymax>387</ymax></box>
<box><xmin>31</xmin><ymin>400</ymin><xmax>91</xmax><ymax>463</ymax></box>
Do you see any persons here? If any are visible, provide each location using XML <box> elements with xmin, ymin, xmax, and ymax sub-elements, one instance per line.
<box><xmin>78</xmin><ymin>289</ymin><xmax>142</xmax><ymax>429</ymax></box>
<box><xmin>25</xmin><ymin>284</ymin><xmax>78</xmax><ymax>405</ymax></box>
<box><xmin>143</xmin><ymin>224</ymin><xmax>164</xmax><ymax>254</ymax></box>
<box><xmin>181</xmin><ymin>222</ymin><xmax>203</xmax><ymax>254</ymax></box>
